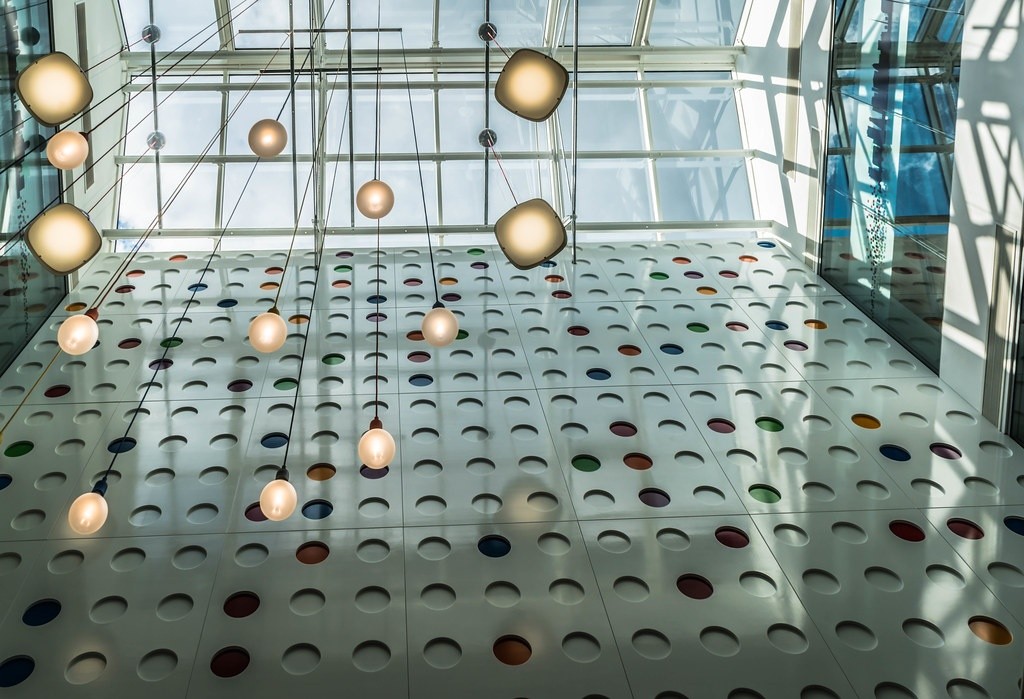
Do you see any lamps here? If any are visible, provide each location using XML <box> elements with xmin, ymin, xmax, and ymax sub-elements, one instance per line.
<box><xmin>478</xmin><ymin>21</ymin><xmax>570</xmax><ymax>123</ymax></box>
<box><xmin>25</xmin><ymin>132</ymin><xmax>166</xmax><ymax>277</ymax></box>
<box><xmin>17</xmin><ymin>24</ymin><xmax>161</xmax><ymax>129</ymax></box>
<box><xmin>477</xmin><ymin>128</ymin><xmax>569</xmax><ymax>271</ymax></box>
